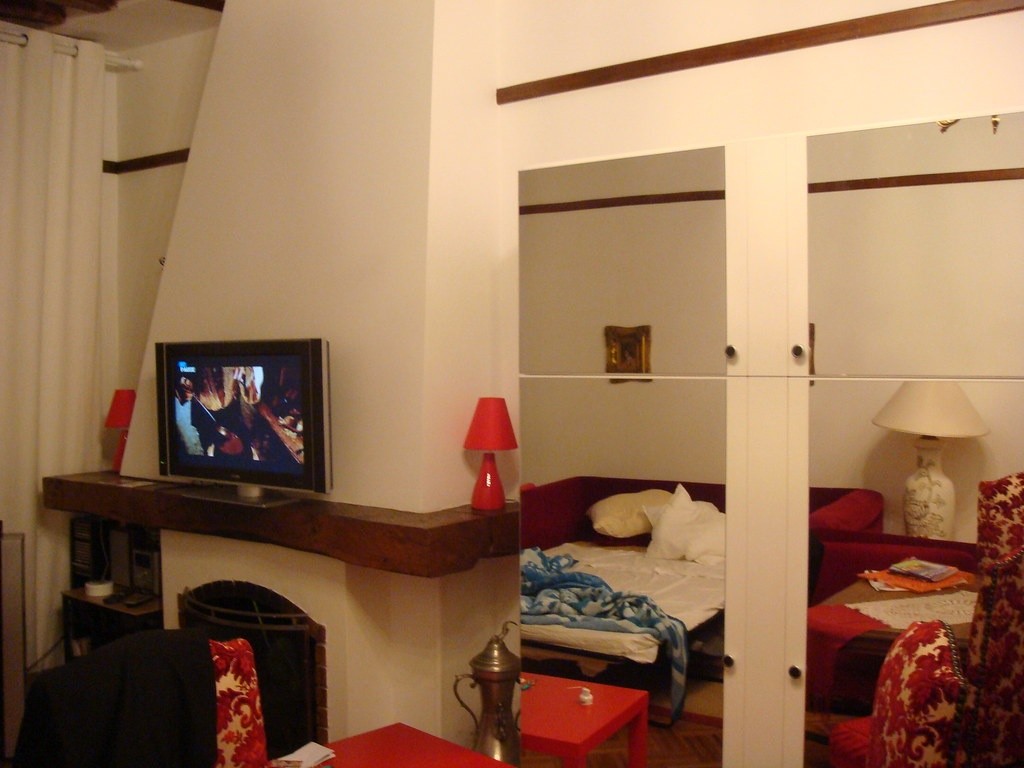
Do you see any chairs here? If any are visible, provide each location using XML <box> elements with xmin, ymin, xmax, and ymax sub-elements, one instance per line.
<box><xmin>11</xmin><ymin>628</ymin><xmax>308</xmax><ymax>768</ymax></box>
<box><xmin>833</xmin><ymin>472</ymin><xmax>1024</xmax><ymax>768</ymax></box>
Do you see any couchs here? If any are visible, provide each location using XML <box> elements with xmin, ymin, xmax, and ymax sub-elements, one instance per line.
<box><xmin>521</xmin><ymin>476</ymin><xmax>884</xmax><ymax>680</ymax></box>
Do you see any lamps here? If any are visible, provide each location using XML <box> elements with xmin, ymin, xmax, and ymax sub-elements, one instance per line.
<box><xmin>872</xmin><ymin>381</ymin><xmax>990</xmax><ymax>539</ymax></box>
<box><xmin>105</xmin><ymin>389</ymin><xmax>135</xmax><ymax>472</ymax></box>
<box><xmin>463</xmin><ymin>397</ymin><xmax>518</xmax><ymax>511</ymax></box>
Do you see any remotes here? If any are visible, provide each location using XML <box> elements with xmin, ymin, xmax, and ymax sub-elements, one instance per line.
<box><xmin>102</xmin><ymin>587</ymin><xmax>157</xmax><ymax>608</ymax></box>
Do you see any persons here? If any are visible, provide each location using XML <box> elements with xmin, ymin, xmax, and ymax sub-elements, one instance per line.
<box><xmin>173</xmin><ymin>356</ymin><xmax>306</xmax><ymax>475</ymax></box>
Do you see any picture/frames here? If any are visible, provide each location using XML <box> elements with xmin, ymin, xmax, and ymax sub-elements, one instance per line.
<box><xmin>603</xmin><ymin>325</ymin><xmax>653</xmax><ymax>384</ymax></box>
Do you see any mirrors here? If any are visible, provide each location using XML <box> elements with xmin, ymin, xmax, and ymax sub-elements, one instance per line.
<box><xmin>806</xmin><ymin>113</ymin><xmax>1024</xmax><ymax>767</ymax></box>
<box><xmin>519</xmin><ymin>147</ymin><xmax>727</xmax><ymax>768</ymax></box>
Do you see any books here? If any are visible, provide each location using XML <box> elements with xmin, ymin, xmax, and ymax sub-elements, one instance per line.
<box><xmin>889</xmin><ymin>560</ymin><xmax>959</xmax><ymax>583</ymax></box>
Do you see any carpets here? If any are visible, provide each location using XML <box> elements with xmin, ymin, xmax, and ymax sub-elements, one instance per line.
<box><xmin>520</xmin><ymin>706</ymin><xmax>832</xmax><ymax>768</ymax></box>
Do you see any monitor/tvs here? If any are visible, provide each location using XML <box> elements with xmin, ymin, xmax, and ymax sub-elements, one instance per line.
<box><xmin>154</xmin><ymin>338</ymin><xmax>333</xmax><ymax>507</ymax></box>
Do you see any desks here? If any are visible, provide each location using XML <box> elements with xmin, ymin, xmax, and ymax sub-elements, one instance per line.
<box><xmin>60</xmin><ymin>587</ymin><xmax>163</xmax><ymax>664</ymax></box>
<box><xmin>808</xmin><ymin>569</ymin><xmax>982</xmax><ymax>717</ymax></box>
<box><xmin>314</xmin><ymin>722</ymin><xmax>517</xmax><ymax>768</ymax></box>
<box><xmin>519</xmin><ymin>672</ymin><xmax>650</xmax><ymax>768</ymax></box>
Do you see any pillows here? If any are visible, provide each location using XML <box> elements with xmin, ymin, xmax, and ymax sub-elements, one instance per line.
<box><xmin>867</xmin><ymin>620</ymin><xmax>978</xmax><ymax>768</ymax></box>
<box><xmin>586</xmin><ymin>489</ymin><xmax>726</xmax><ymax>564</ymax></box>
